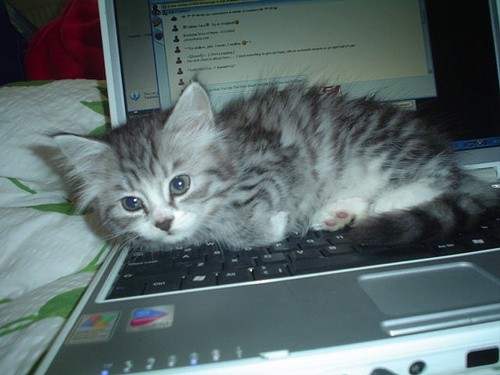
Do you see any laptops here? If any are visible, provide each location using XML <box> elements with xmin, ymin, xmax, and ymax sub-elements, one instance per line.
<box><xmin>29</xmin><ymin>0</ymin><xmax>500</xmax><ymax>375</ymax></box>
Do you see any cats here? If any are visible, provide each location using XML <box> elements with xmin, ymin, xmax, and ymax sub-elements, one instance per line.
<box><xmin>53</xmin><ymin>62</ymin><xmax>495</xmax><ymax>251</ymax></box>
